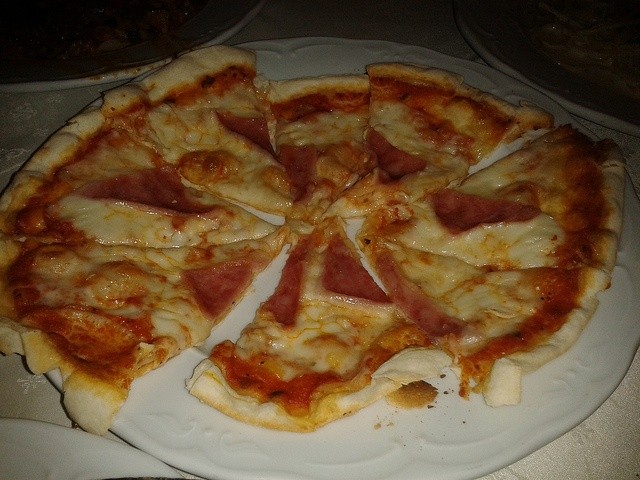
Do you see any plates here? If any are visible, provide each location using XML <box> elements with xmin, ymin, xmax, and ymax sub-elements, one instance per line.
<box><xmin>460</xmin><ymin>1</ymin><xmax>639</xmax><ymax>138</ymax></box>
<box><xmin>2</xmin><ymin>2</ymin><xmax>261</xmax><ymax>92</ymax></box>
<box><xmin>3</xmin><ymin>415</ymin><xmax>186</xmax><ymax>478</ymax></box>
